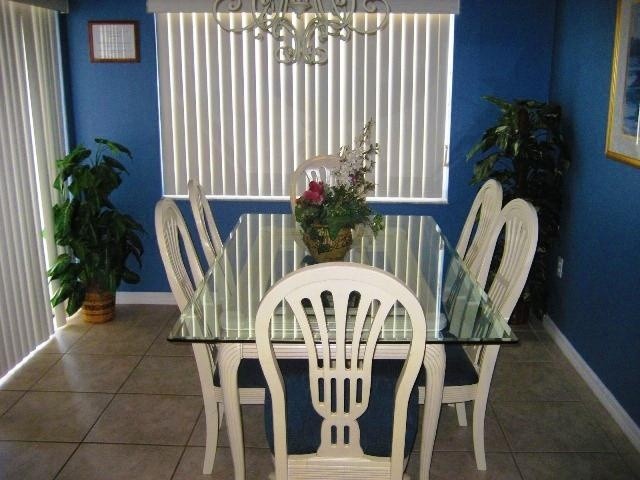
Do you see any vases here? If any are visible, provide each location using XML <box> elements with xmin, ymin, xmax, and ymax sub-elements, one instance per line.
<box><xmin>303</xmin><ymin>224</ymin><xmax>353</xmax><ymax>263</ymax></box>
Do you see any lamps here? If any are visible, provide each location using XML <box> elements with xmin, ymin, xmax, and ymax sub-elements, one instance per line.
<box><xmin>212</xmin><ymin>0</ymin><xmax>390</xmax><ymax>65</ymax></box>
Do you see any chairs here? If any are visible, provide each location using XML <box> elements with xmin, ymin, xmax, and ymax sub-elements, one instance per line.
<box><xmin>442</xmin><ymin>178</ymin><xmax>503</xmax><ymax>428</ymax></box>
<box><xmin>188</xmin><ymin>180</ymin><xmax>235</xmax><ymax>305</ymax></box>
<box><xmin>254</xmin><ymin>262</ymin><xmax>426</xmax><ymax>480</ymax></box>
<box><xmin>417</xmin><ymin>197</ymin><xmax>539</xmax><ymax>471</ymax></box>
<box><xmin>155</xmin><ymin>198</ymin><xmax>267</xmax><ymax>476</ymax></box>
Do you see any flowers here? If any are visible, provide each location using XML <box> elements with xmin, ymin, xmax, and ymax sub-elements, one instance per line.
<box><xmin>290</xmin><ymin>117</ymin><xmax>385</xmax><ymax>254</ymax></box>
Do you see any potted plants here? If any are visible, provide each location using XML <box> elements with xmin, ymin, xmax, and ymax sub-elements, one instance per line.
<box><xmin>466</xmin><ymin>96</ymin><xmax>571</xmax><ymax>325</ymax></box>
<box><xmin>46</xmin><ymin>138</ymin><xmax>145</xmax><ymax>324</ymax></box>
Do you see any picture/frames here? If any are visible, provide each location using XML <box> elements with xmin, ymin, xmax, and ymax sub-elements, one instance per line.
<box><xmin>605</xmin><ymin>0</ymin><xmax>639</xmax><ymax>166</ymax></box>
<box><xmin>88</xmin><ymin>19</ymin><xmax>140</xmax><ymax>62</ymax></box>
